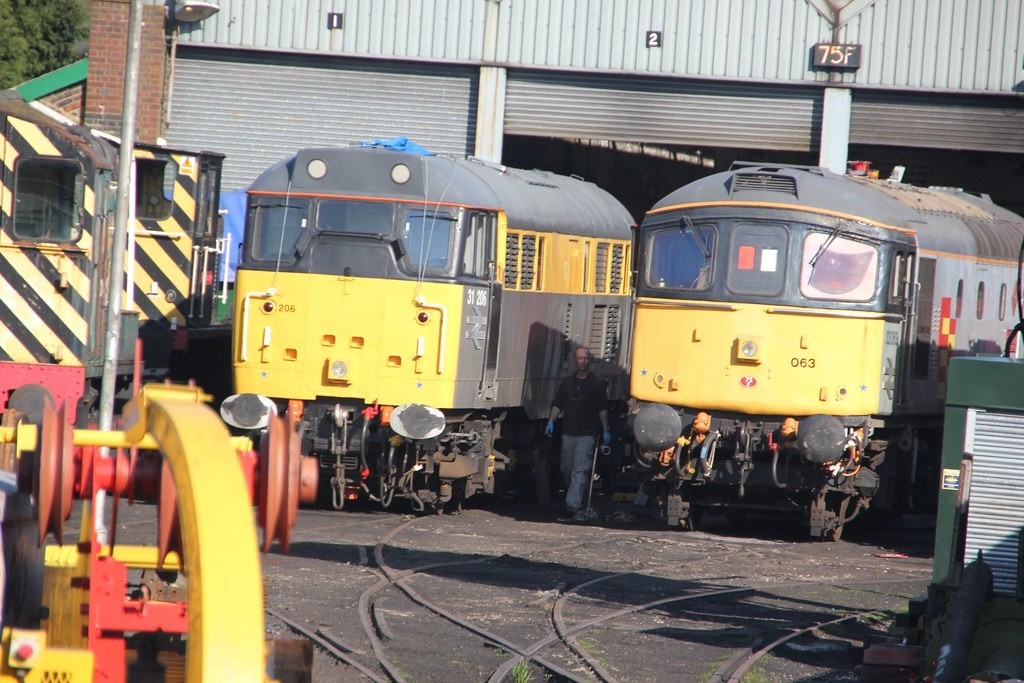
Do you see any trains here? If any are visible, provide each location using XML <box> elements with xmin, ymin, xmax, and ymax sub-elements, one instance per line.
<box><xmin>630</xmin><ymin>162</ymin><xmax>1024</xmax><ymax>544</ymax></box>
<box><xmin>230</xmin><ymin>142</ymin><xmax>640</xmax><ymax>514</ymax></box>
<box><xmin>1</xmin><ymin>90</ymin><xmax>320</xmax><ymax>680</ymax></box>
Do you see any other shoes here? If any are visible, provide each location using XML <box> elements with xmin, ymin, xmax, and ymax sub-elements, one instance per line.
<box><xmin>556</xmin><ymin>514</ymin><xmax>571</xmax><ymax>522</ymax></box>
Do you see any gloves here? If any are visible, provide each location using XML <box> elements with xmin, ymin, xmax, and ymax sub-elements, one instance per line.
<box><xmin>544</xmin><ymin>421</ymin><xmax>553</xmax><ymax>436</ymax></box>
<box><xmin>603</xmin><ymin>431</ymin><xmax>610</xmax><ymax>446</ymax></box>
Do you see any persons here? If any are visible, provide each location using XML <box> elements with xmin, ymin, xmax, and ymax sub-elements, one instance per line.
<box><xmin>544</xmin><ymin>347</ymin><xmax>611</xmax><ymax>522</ymax></box>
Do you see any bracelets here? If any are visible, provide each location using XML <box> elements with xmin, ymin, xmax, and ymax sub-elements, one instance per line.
<box><xmin>602</xmin><ymin>426</ymin><xmax>609</xmax><ymax>431</ymax></box>
